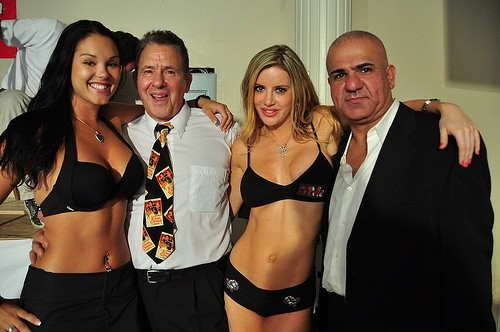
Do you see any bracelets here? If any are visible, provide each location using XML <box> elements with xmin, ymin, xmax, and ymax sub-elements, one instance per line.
<box><xmin>193</xmin><ymin>95</ymin><xmax>211</xmax><ymax>108</ymax></box>
<box><xmin>421</xmin><ymin>98</ymin><xmax>442</xmax><ymax>112</ymax></box>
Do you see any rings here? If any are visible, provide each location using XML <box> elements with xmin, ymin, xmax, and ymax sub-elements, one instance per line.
<box><xmin>8</xmin><ymin>325</ymin><xmax>16</xmax><ymax>331</ymax></box>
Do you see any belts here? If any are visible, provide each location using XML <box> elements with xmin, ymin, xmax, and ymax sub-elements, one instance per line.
<box><xmin>139</xmin><ymin>264</ymin><xmax>207</xmax><ymax>284</ymax></box>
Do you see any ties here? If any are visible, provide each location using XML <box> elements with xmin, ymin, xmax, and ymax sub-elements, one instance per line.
<box><xmin>142</xmin><ymin>123</ymin><xmax>178</xmax><ymax>265</ymax></box>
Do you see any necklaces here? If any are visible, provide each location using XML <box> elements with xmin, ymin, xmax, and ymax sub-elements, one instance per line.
<box><xmin>264</xmin><ymin>125</ymin><xmax>296</xmax><ymax>157</ymax></box>
<box><xmin>70</xmin><ymin>109</ymin><xmax>105</xmax><ymax>144</ymax></box>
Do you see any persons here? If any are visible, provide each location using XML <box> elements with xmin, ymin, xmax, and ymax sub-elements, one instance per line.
<box><xmin>31</xmin><ymin>31</ymin><xmax>241</xmax><ymax>332</ymax></box>
<box><xmin>0</xmin><ymin>16</ymin><xmax>139</xmax><ymax>226</ymax></box>
<box><xmin>309</xmin><ymin>31</ymin><xmax>496</xmax><ymax>332</ymax></box>
<box><xmin>2</xmin><ymin>20</ymin><xmax>232</xmax><ymax>332</ymax></box>
<box><xmin>226</xmin><ymin>45</ymin><xmax>480</xmax><ymax>332</ymax></box>
